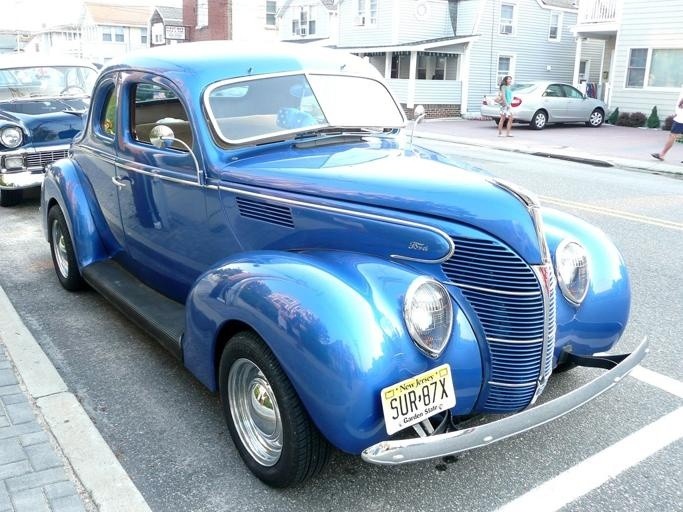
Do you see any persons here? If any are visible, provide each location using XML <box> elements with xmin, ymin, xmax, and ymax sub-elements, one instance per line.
<box><xmin>496</xmin><ymin>76</ymin><xmax>515</xmax><ymax>136</ymax></box>
<box><xmin>651</xmin><ymin>86</ymin><xmax>683</xmax><ymax>163</ymax></box>
<box><xmin>432</xmin><ymin>71</ymin><xmax>443</xmax><ymax>79</ymax></box>
<box><xmin>600</xmin><ymin>71</ymin><xmax>609</xmax><ymax>101</ymax></box>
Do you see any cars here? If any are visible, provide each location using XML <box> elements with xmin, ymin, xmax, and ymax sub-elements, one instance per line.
<box><xmin>0</xmin><ymin>60</ymin><xmax>97</xmax><ymax>207</ymax></box>
<box><xmin>480</xmin><ymin>80</ymin><xmax>608</xmax><ymax>130</ymax></box>
<box><xmin>40</xmin><ymin>41</ymin><xmax>648</xmax><ymax>486</ymax></box>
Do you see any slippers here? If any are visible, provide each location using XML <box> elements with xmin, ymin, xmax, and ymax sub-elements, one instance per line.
<box><xmin>651</xmin><ymin>153</ymin><xmax>664</xmax><ymax>161</ymax></box>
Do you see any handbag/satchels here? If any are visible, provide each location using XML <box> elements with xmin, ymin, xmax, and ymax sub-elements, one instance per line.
<box><xmin>495</xmin><ymin>91</ymin><xmax>505</xmax><ymax>107</ymax></box>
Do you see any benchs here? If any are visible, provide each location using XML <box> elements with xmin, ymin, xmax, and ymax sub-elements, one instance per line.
<box><xmin>136</xmin><ymin>115</ymin><xmax>282</xmax><ymax>150</ymax></box>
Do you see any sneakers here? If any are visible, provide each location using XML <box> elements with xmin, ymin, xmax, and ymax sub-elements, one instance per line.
<box><xmin>498</xmin><ymin>133</ymin><xmax>513</xmax><ymax>136</ymax></box>
